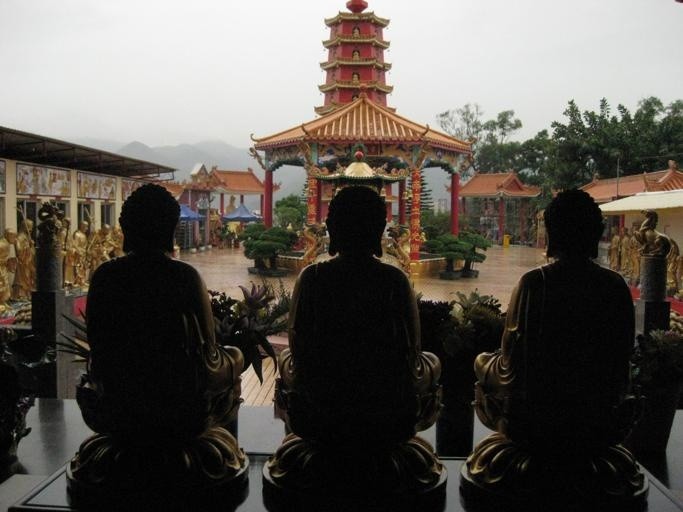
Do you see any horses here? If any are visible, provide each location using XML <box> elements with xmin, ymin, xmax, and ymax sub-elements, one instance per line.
<box><xmin>633</xmin><ymin>210</ymin><xmax>672</xmax><ymax>258</ymax></box>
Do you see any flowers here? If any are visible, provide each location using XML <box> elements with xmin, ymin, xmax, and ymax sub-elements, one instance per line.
<box><xmin>45</xmin><ymin>271</ymin><xmax>291</xmax><ymax>386</ymax></box>
<box><xmin>411</xmin><ymin>281</ymin><xmax>507</xmax><ymax>384</ymax></box>
<box><xmin>631</xmin><ymin>329</ymin><xmax>683</xmax><ymax>397</ymax></box>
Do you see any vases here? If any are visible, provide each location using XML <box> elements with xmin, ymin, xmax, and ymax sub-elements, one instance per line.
<box><xmin>219</xmin><ymin>378</ymin><xmax>242</xmax><ymax>443</ymax></box>
<box><xmin>436</xmin><ymin>361</ymin><xmax>477</xmax><ymax>461</ymax></box>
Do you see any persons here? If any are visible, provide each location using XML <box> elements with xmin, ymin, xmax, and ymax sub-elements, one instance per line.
<box><xmin>605</xmin><ymin>222</ymin><xmax>682</xmax><ymax>290</ymax></box>
<box><xmin>62</xmin><ymin>181</ymin><xmax>255</xmax><ymax>459</ymax></box>
<box><xmin>266</xmin><ymin>183</ymin><xmax>447</xmax><ymax>447</ymax></box>
<box><xmin>468</xmin><ymin>185</ymin><xmax>639</xmax><ymax>454</ymax></box>
<box><xmin>0</xmin><ymin>212</ymin><xmax>124</xmax><ymax>306</ymax></box>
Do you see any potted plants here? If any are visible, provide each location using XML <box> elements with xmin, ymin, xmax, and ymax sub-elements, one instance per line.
<box><xmin>425</xmin><ymin>232</ymin><xmax>492</xmax><ymax>280</ymax></box>
<box><xmin>238</xmin><ymin>224</ymin><xmax>298</xmax><ymax>277</ymax></box>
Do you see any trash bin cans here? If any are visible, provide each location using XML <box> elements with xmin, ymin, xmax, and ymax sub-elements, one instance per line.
<box><xmin>503</xmin><ymin>235</ymin><xmax>510</xmax><ymax>248</ymax></box>
<box><xmin>234</xmin><ymin>239</ymin><xmax>240</xmax><ymax>248</ymax></box>
